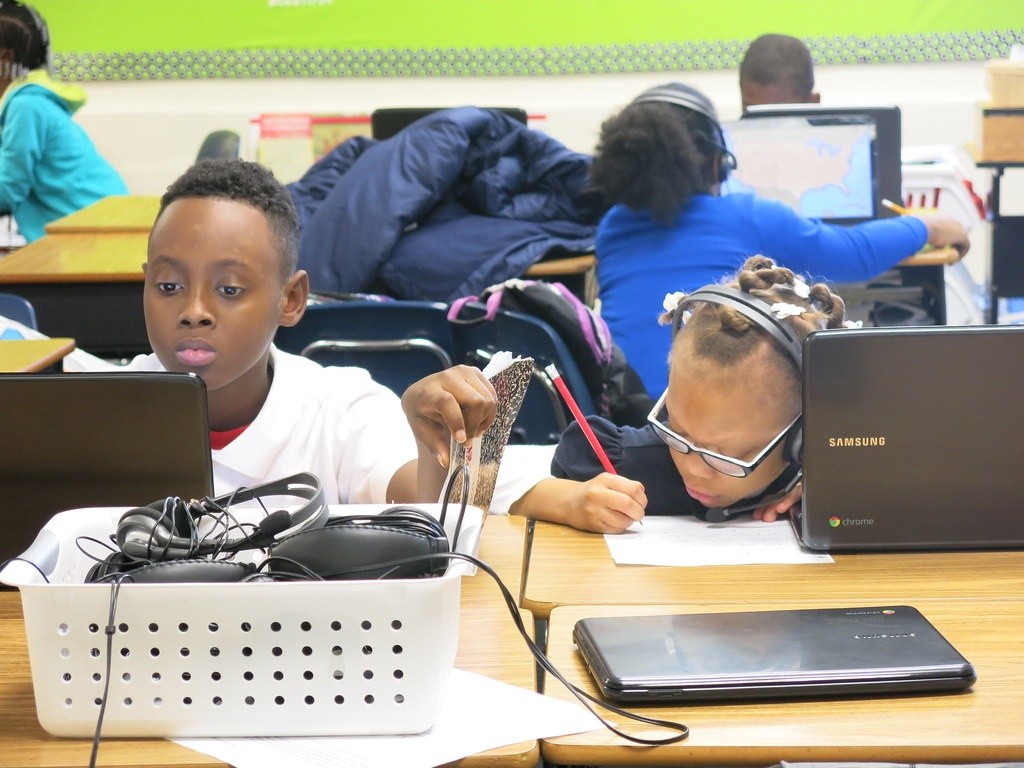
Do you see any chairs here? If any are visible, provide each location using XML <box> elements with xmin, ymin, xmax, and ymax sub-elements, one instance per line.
<box><xmin>272</xmin><ymin>302</ymin><xmax>457</xmax><ymax>398</ymax></box>
<box><xmin>452</xmin><ymin>298</ymin><xmax>598</xmax><ymax>445</ymax></box>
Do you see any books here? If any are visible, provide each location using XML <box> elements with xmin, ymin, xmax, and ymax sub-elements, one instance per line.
<box><xmin>447</xmin><ymin>350</ymin><xmax>534</xmax><ymax>532</ymax></box>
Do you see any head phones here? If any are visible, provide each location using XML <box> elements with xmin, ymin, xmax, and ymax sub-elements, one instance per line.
<box><xmin>628</xmin><ymin>92</ymin><xmax>737</xmax><ymax>182</ymax></box>
<box><xmin>83</xmin><ymin>471</ymin><xmax>448</xmax><ymax>586</ymax></box>
<box><xmin>670</xmin><ymin>285</ymin><xmax>805</xmax><ymax>466</ymax></box>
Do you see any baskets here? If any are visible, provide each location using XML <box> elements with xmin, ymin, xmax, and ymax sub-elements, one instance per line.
<box><xmin>0</xmin><ymin>503</ymin><xmax>484</xmax><ymax>738</ymax></box>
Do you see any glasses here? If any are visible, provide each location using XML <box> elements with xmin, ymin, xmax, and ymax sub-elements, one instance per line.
<box><xmin>647</xmin><ymin>385</ymin><xmax>803</xmax><ymax>477</ymax></box>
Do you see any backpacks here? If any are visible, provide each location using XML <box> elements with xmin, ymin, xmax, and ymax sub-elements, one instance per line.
<box><xmin>446</xmin><ymin>278</ymin><xmax>666</xmax><ymax>429</ymax></box>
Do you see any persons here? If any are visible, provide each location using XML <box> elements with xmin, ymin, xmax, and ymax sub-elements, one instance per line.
<box><xmin>1</xmin><ymin>0</ymin><xmax>131</xmax><ymax>245</ymax></box>
<box><xmin>739</xmin><ymin>33</ymin><xmax>821</xmax><ymax>116</ymax></box>
<box><xmin>488</xmin><ymin>253</ymin><xmax>862</xmax><ymax>536</ymax></box>
<box><xmin>102</xmin><ymin>159</ymin><xmax>497</xmax><ymax>505</ymax></box>
<box><xmin>593</xmin><ymin>82</ymin><xmax>972</xmax><ymax>403</ymax></box>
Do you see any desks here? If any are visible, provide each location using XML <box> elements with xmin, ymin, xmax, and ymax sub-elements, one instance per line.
<box><xmin>0</xmin><ymin>514</ymin><xmax>1024</xmax><ymax>768</ymax></box>
<box><xmin>0</xmin><ymin>195</ymin><xmax>162</xmax><ymax>358</ymax></box>
<box><xmin>966</xmin><ymin>143</ymin><xmax>1024</xmax><ymax>325</ymax></box>
<box><xmin>0</xmin><ymin>338</ymin><xmax>75</xmax><ymax>373</ymax></box>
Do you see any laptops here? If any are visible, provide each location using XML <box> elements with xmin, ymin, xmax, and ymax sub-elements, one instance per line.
<box><xmin>788</xmin><ymin>323</ymin><xmax>1024</xmax><ymax>555</ymax></box>
<box><xmin>571</xmin><ymin>606</ymin><xmax>976</xmax><ymax>701</ymax></box>
<box><xmin>739</xmin><ymin>106</ymin><xmax>904</xmax><ymax>222</ymax></box>
<box><xmin>371</xmin><ymin>107</ymin><xmax>528</xmax><ymax>141</ymax></box>
<box><xmin>715</xmin><ymin>115</ymin><xmax>877</xmax><ymax>229</ymax></box>
<box><xmin>0</xmin><ymin>371</ymin><xmax>216</xmax><ymax>593</ymax></box>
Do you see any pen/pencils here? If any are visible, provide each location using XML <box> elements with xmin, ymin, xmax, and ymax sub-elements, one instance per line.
<box><xmin>545</xmin><ymin>363</ymin><xmax>643</xmax><ymax>524</ymax></box>
<box><xmin>881</xmin><ymin>197</ymin><xmax>907</xmax><ymax>215</ymax></box>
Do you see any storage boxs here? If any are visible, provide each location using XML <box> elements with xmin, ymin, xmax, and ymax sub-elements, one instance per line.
<box><xmin>976</xmin><ymin>102</ymin><xmax>1024</xmax><ymax>159</ymax></box>
<box><xmin>0</xmin><ymin>504</ymin><xmax>486</xmax><ymax>737</ymax></box>
<box><xmin>988</xmin><ymin>60</ymin><xmax>1024</xmax><ymax>106</ymax></box>
